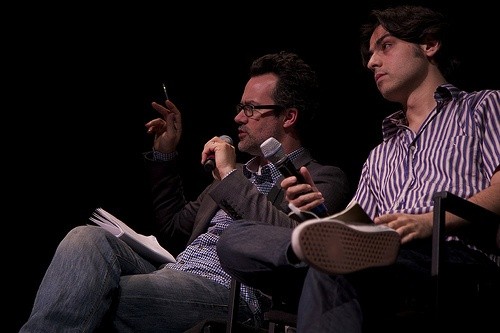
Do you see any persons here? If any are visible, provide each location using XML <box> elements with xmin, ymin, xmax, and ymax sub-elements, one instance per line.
<box><xmin>18</xmin><ymin>50</ymin><xmax>348</xmax><ymax>333</ymax></box>
<box><xmin>216</xmin><ymin>6</ymin><xmax>500</xmax><ymax>333</ymax></box>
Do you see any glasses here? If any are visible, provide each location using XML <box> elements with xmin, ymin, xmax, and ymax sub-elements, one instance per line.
<box><xmin>236</xmin><ymin>101</ymin><xmax>288</xmax><ymax>118</ymax></box>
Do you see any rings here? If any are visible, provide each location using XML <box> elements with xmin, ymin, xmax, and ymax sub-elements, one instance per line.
<box><xmin>213</xmin><ymin>141</ymin><xmax>215</xmax><ymax>144</ymax></box>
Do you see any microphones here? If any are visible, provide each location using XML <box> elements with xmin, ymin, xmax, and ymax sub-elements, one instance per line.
<box><xmin>204</xmin><ymin>135</ymin><xmax>233</xmax><ymax>171</ymax></box>
<box><xmin>260</xmin><ymin>137</ymin><xmax>330</xmax><ymax>218</ymax></box>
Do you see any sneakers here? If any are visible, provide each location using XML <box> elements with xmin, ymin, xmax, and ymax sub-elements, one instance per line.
<box><xmin>292</xmin><ymin>213</ymin><xmax>401</xmax><ymax>273</ymax></box>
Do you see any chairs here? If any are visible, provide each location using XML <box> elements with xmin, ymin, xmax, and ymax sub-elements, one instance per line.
<box><xmin>226</xmin><ymin>190</ymin><xmax>500</xmax><ymax>333</ymax></box>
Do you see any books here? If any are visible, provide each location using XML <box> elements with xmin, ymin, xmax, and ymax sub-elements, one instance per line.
<box><xmin>86</xmin><ymin>207</ymin><xmax>176</xmax><ymax>265</ymax></box>
